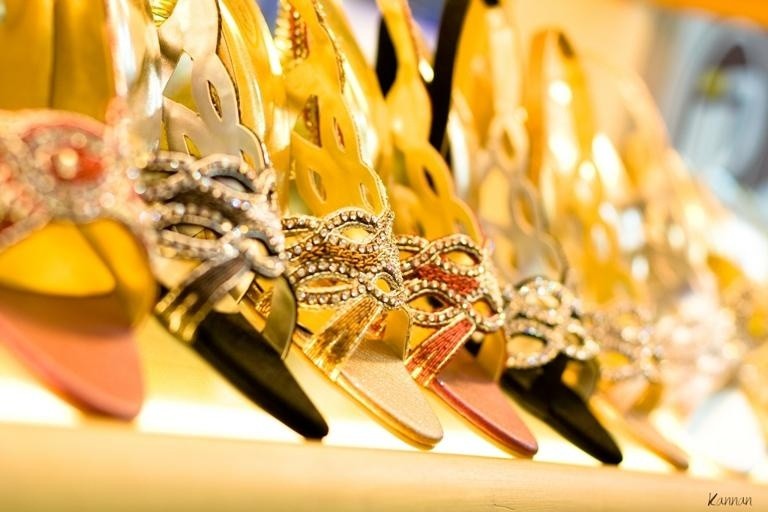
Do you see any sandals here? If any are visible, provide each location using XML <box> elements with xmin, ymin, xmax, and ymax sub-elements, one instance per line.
<box><xmin>453</xmin><ymin>0</ymin><xmax>691</xmax><ymax>472</ymax></box>
<box><xmin>2</xmin><ymin>0</ymin><xmax>158</xmax><ymax>423</ymax></box>
<box><xmin>377</xmin><ymin>0</ymin><xmax>623</xmax><ymax>465</ymax></box>
<box><xmin>290</xmin><ymin>0</ymin><xmax>538</xmax><ymax>461</ymax></box>
<box><xmin>126</xmin><ymin>0</ymin><xmax>328</xmax><ymax>440</ymax></box>
<box><xmin>203</xmin><ymin>0</ymin><xmax>444</xmax><ymax>451</ymax></box>
